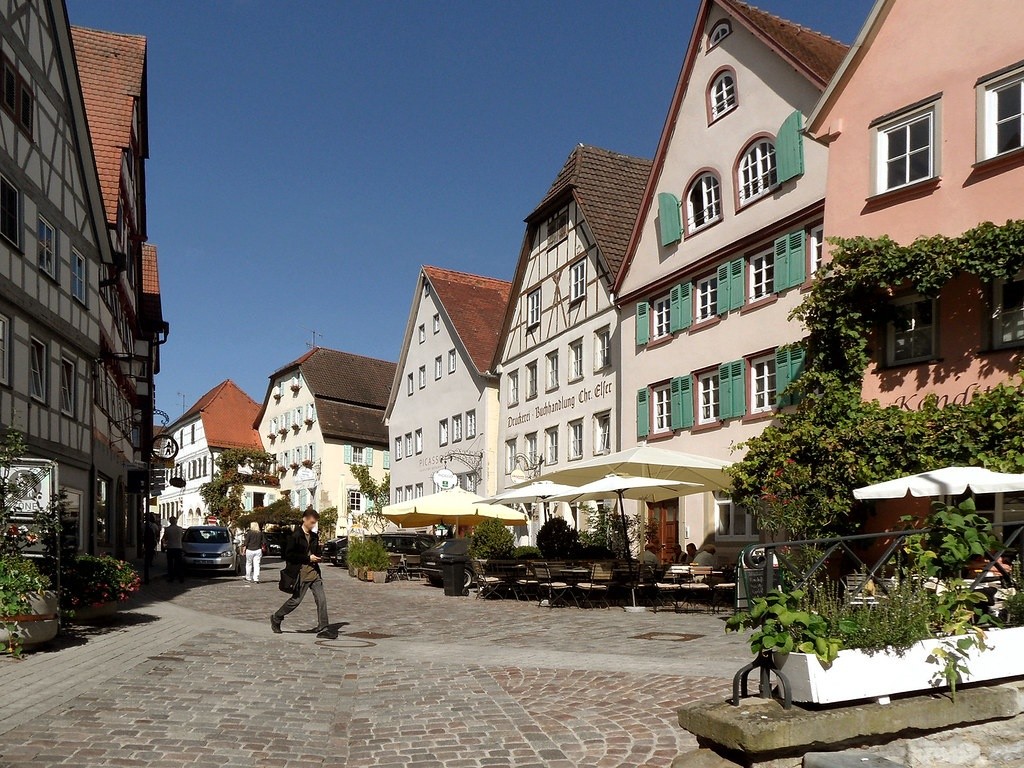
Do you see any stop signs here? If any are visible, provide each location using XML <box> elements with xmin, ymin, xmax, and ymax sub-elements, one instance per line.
<box><xmin>208</xmin><ymin>516</ymin><xmax>217</xmax><ymax>524</ymax></box>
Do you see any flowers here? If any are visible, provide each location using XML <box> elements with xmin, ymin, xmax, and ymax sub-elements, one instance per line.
<box><xmin>290</xmin><ymin>384</ymin><xmax>302</xmax><ymax>393</ymax></box>
<box><xmin>302</xmin><ymin>460</ymin><xmax>313</xmax><ymax>467</ymax></box>
<box><xmin>291</xmin><ymin>423</ymin><xmax>301</xmax><ymax>432</ymax></box>
<box><xmin>276</xmin><ymin>465</ymin><xmax>288</xmax><ymax>474</ymax></box>
<box><xmin>277</xmin><ymin>428</ymin><xmax>289</xmax><ymax>436</ymax></box>
<box><xmin>0</xmin><ymin>522</ymin><xmax>59</xmax><ymax>661</ymax></box>
<box><xmin>60</xmin><ymin>554</ymin><xmax>142</xmax><ymax>609</ymax></box>
<box><xmin>304</xmin><ymin>418</ymin><xmax>317</xmax><ymax>427</ymax></box>
<box><xmin>290</xmin><ymin>462</ymin><xmax>300</xmax><ymax>476</ymax></box>
<box><xmin>273</xmin><ymin>394</ymin><xmax>281</xmax><ymax>401</ymax></box>
<box><xmin>266</xmin><ymin>433</ymin><xmax>277</xmax><ymax>440</ymax></box>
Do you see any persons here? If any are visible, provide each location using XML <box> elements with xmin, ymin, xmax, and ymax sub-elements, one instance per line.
<box><xmin>636</xmin><ymin>544</ymin><xmax>658</xmax><ymax>564</ymax></box>
<box><xmin>670</xmin><ymin>544</ymin><xmax>687</xmax><ymax>564</ymax></box>
<box><xmin>161</xmin><ymin>516</ymin><xmax>186</xmax><ymax>584</ymax></box>
<box><xmin>141</xmin><ymin>512</ymin><xmax>159</xmax><ymax>559</ymax></box>
<box><xmin>270</xmin><ymin>509</ymin><xmax>338</xmax><ymax>639</ymax></box>
<box><xmin>693</xmin><ymin>544</ymin><xmax>719</xmax><ymax>566</ymax></box>
<box><xmin>241</xmin><ymin>522</ymin><xmax>264</xmax><ymax>583</ymax></box>
<box><xmin>681</xmin><ymin>543</ymin><xmax>698</xmax><ymax>564</ymax></box>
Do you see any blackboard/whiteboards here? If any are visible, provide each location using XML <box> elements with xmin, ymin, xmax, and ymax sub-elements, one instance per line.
<box><xmin>736</xmin><ymin>543</ymin><xmax>787</xmax><ymax>612</ymax></box>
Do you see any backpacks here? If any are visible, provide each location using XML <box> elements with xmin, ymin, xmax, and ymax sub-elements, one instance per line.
<box><xmin>143</xmin><ymin>523</ymin><xmax>157</xmax><ymax>550</ymax></box>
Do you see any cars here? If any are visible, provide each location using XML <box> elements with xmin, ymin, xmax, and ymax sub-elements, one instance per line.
<box><xmin>182</xmin><ymin>525</ymin><xmax>240</xmax><ymax>575</ymax></box>
<box><xmin>366</xmin><ymin>532</ymin><xmax>442</xmax><ymax>572</ymax></box>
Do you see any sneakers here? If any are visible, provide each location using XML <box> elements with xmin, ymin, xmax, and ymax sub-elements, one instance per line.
<box><xmin>270</xmin><ymin>615</ymin><xmax>282</xmax><ymax>633</ymax></box>
<box><xmin>317</xmin><ymin>628</ymin><xmax>338</xmax><ymax>638</ymax></box>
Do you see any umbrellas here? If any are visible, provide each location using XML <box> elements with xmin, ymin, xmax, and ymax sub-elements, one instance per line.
<box><xmin>853</xmin><ymin>467</ymin><xmax>1024</xmax><ymax>500</ymax></box>
<box><xmin>382</xmin><ymin>439</ymin><xmax>740</xmax><ymax>562</ymax></box>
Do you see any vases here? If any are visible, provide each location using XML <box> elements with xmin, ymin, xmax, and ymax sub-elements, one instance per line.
<box><xmin>61</xmin><ymin>601</ymin><xmax>117</xmax><ymax>620</ymax></box>
<box><xmin>0</xmin><ymin>591</ymin><xmax>58</xmax><ymax>651</ymax></box>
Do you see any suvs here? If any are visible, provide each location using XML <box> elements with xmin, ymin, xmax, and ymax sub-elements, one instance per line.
<box><xmin>420</xmin><ymin>538</ymin><xmax>503</xmax><ymax>588</ymax></box>
<box><xmin>320</xmin><ymin>535</ymin><xmax>348</xmax><ymax>567</ymax></box>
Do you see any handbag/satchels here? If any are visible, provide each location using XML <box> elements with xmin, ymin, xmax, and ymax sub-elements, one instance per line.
<box><xmin>278</xmin><ymin>570</ymin><xmax>300</xmax><ymax>593</ymax></box>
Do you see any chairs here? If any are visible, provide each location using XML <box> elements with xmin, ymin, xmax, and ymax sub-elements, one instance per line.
<box><xmin>385</xmin><ymin>554</ymin><xmax>425</xmax><ymax>582</ymax></box>
<box><xmin>466</xmin><ymin>560</ymin><xmax>740</xmax><ymax>614</ymax></box>
<box><xmin>841</xmin><ymin>574</ymin><xmax>895</xmax><ymax>615</ymax></box>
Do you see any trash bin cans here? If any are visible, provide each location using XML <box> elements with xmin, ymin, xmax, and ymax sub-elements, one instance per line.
<box><xmin>439</xmin><ymin>553</ymin><xmax>469</xmax><ymax>597</ymax></box>
<box><xmin>341</xmin><ymin>549</ymin><xmax>348</xmax><ymax>569</ymax></box>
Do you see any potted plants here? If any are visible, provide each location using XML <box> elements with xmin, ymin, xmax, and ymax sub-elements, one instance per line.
<box><xmin>346</xmin><ymin>534</ymin><xmax>391</xmax><ymax>583</ymax></box>
<box><xmin>724</xmin><ymin>497</ymin><xmax>1024</xmax><ymax>705</ymax></box>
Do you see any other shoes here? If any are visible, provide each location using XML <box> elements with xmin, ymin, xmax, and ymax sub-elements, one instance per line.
<box><xmin>243</xmin><ymin>578</ymin><xmax>251</xmax><ymax>582</ymax></box>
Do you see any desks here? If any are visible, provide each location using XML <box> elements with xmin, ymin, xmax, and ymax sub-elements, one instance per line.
<box><xmin>495</xmin><ymin>565</ymin><xmax>531</xmax><ymax>604</ymax></box>
<box><xmin>560</xmin><ymin>570</ymin><xmax>591</xmax><ymax>606</ymax></box>
